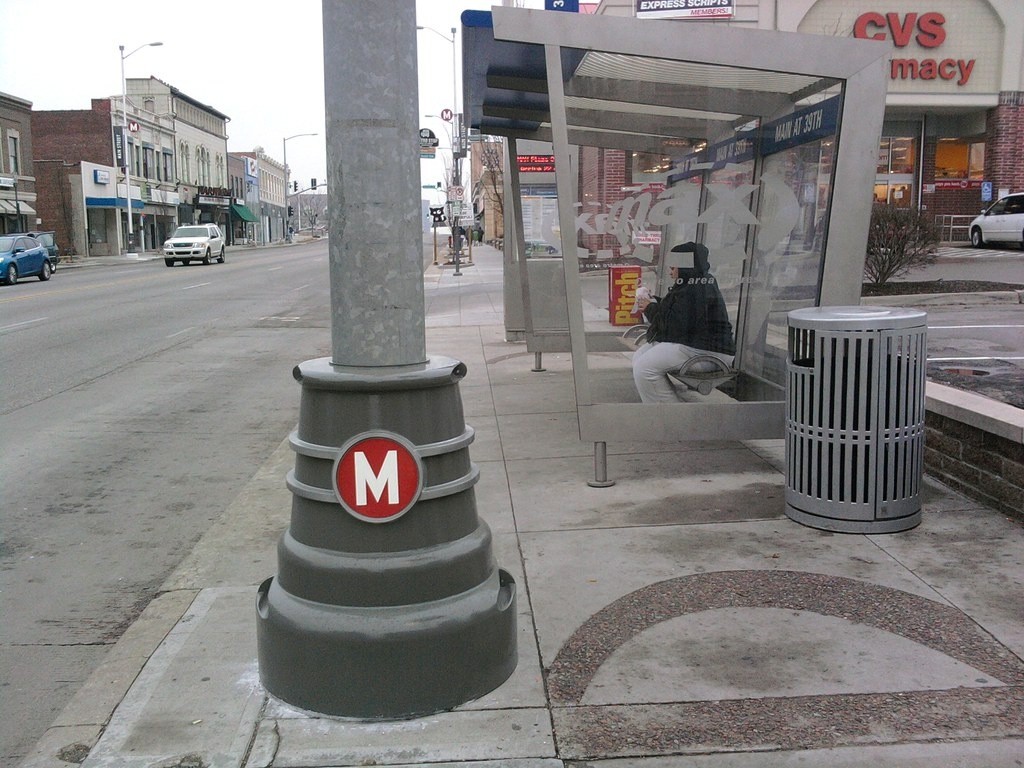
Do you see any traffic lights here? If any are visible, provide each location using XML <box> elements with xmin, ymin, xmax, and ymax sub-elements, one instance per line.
<box><xmin>294</xmin><ymin>181</ymin><xmax>298</xmax><ymax>192</ymax></box>
<box><xmin>288</xmin><ymin>206</ymin><xmax>294</xmax><ymax>217</ymax></box>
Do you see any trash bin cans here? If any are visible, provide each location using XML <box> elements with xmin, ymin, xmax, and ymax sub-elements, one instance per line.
<box><xmin>786</xmin><ymin>306</ymin><xmax>928</xmax><ymax>534</ymax></box>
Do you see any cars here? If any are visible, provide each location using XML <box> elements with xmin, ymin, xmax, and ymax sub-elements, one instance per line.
<box><xmin>0</xmin><ymin>230</ymin><xmax>59</xmax><ymax>274</ymax></box>
<box><xmin>0</xmin><ymin>236</ymin><xmax>51</xmax><ymax>285</ymax></box>
<box><xmin>968</xmin><ymin>192</ymin><xmax>1024</xmax><ymax>249</ymax></box>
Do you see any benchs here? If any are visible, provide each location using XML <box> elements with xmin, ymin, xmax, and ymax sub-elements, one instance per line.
<box><xmin>617</xmin><ymin>324</ymin><xmax>736</xmax><ymax>396</ymax></box>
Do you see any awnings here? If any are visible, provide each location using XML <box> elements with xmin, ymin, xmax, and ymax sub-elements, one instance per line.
<box><xmin>231</xmin><ymin>204</ymin><xmax>260</xmax><ymax>222</ymax></box>
<box><xmin>0</xmin><ymin>199</ymin><xmax>36</xmax><ymax>214</ymax></box>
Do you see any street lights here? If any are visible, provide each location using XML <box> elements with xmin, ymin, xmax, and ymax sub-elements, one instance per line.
<box><xmin>416</xmin><ymin>25</ymin><xmax>460</xmax><ymax>276</ymax></box>
<box><xmin>119</xmin><ymin>43</ymin><xmax>163</xmax><ymax>252</ymax></box>
<box><xmin>426</xmin><ymin>115</ymin><xmax>454</xmax><ymax>186</ymax></box>
<box><xmin>283</xmin><ymin>133</ymin><xmax>318</xmax><ymax>242</ymax></box>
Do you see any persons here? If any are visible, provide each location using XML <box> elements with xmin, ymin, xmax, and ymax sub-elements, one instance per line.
<box><xmin>873</xmin><ymin>186</ymin><xmax>910</xmax><ymax>207</ymax></box>
<box><xmin>460</xmin><ymin>226</ymin><xmax>484</xmax><ymax>247</ymax></box>
<box><xmin>633</xmin><ymin>242</ymin><xmax>737</xmax><ymax>403</ymax></box>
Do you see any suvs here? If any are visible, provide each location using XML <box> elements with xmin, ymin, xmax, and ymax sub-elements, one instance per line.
<box><xmin>163</xmin><ymin>222</ymin><xmax>226</xmax><ymax>267</ymax></box>
